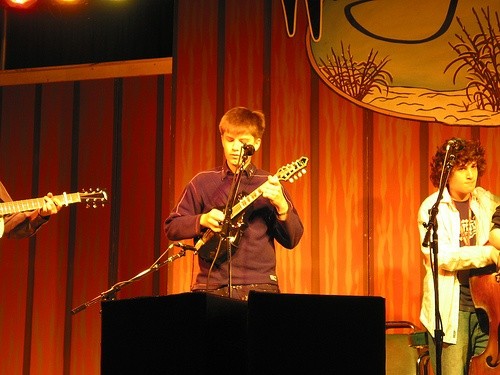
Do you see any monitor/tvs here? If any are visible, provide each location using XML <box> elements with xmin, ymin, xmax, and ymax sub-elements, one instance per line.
<box><xmin>0</xmin><ymin>0</ymin><xmax>177</xmax><ymax>88</ymax></box>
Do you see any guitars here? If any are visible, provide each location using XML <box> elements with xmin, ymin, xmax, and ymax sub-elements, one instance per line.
<box><xmin>0</xmin><ymin>188</ymin><xmax>108</xmax><ymax>239</ymax></box>
<box><xmin>194</xmin><ymin>156</ymin><xmax>309</xmax><ymax>261</ymax></box>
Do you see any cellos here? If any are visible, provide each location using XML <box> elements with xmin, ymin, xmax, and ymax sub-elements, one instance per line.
<box><xmin>468</xmin><ymin>205</ymin><xmax>500</xmax><ymax>375</ymax></box>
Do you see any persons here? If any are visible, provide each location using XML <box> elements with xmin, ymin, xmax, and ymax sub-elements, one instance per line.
<box><xmin>417</xmin><ymin>137</ymin><xmax>500</xmax><ymax>375</ymax></box>
<box><xmin>164</xmin><ymin>106</ymin><xmax>303</xmax><ymax>302</ymax></box>
<box><xmin>488</xmin><ymin>204</ymin><xmax>500</xmax><ymax>250</ymax></box>
<box><xmin>0</xmin><ymin>181</ymin><xmax>62</xmax><ymax>241</ymax></box>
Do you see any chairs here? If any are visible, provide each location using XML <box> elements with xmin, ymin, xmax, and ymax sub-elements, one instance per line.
<box><xmin>386</xmin><ymin>321</ymin><xmax>435</xmax><ymax>375</ymax></box>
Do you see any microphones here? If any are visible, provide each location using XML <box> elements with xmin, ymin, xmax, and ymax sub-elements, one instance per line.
<box><xmin>242</xmin><ymin>145</ymin><xmax>255</xmax><ymax>165</ymax></box>
<box><xmin>448</xmin><ymin>138</ymin><xmax>463</xmax><ymax>151</ymax></box>
